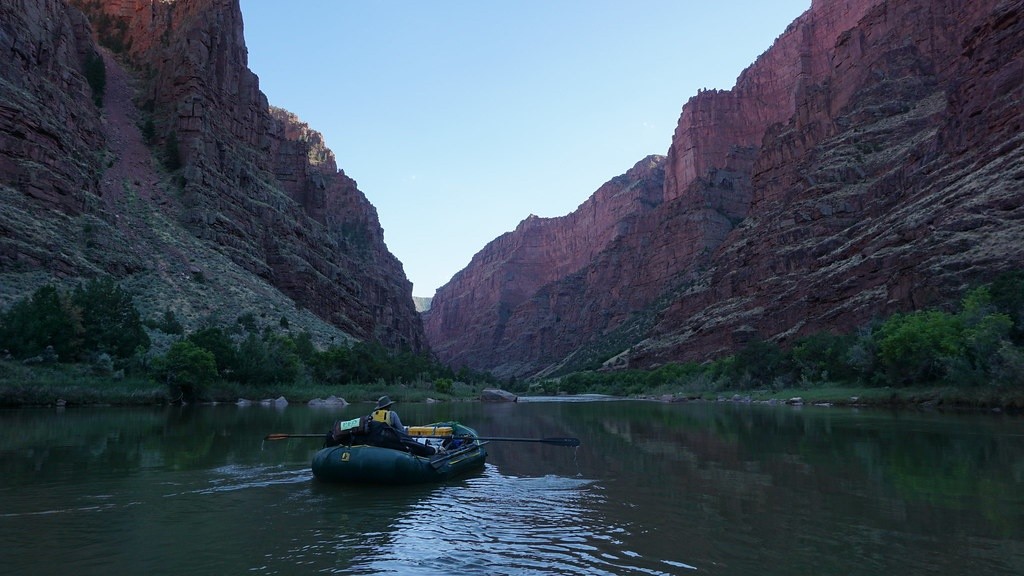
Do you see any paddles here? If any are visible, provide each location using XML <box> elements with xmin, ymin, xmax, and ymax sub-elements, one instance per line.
<box><xmin>263</xmin><ymin>433</ymin><xmax>583</xmax><ymax>448</ymax></box>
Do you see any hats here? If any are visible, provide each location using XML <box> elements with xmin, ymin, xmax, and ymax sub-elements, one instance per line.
<box><xmin>374</xmin><ymin>396</ymin><xmax>396</xmax><ymax>411</ymax></box>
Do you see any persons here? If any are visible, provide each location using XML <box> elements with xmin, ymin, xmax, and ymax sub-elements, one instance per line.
<box><xmin>369</xmin><ymin>395</ymin><xmax>405</xmax><ymax>434</ymax></box>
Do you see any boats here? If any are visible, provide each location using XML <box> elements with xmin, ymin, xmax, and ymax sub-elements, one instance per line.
<box><xmin>310</xmin><ymin>421</ymin><xmax>489</xmax><ymax>487</ymax></box>
